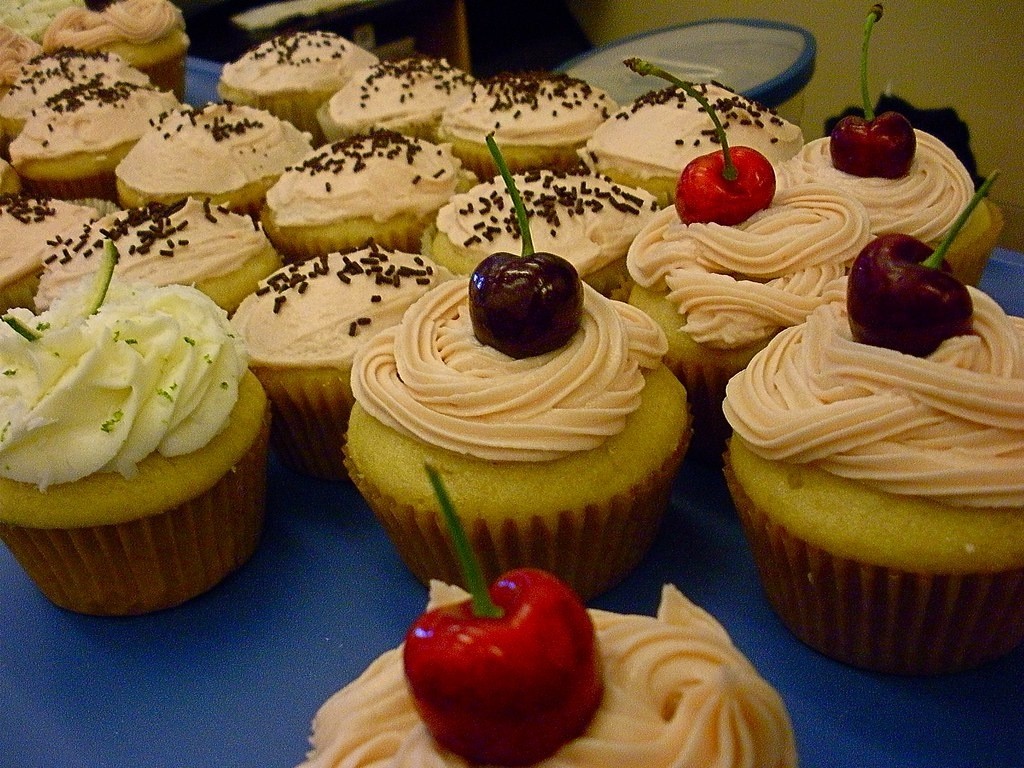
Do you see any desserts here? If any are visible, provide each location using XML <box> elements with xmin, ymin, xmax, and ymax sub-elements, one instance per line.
<box><xmin>0</xmin><ymin>0</ymin><xmax>1024</xmax><ymax>768</ymax></box>
